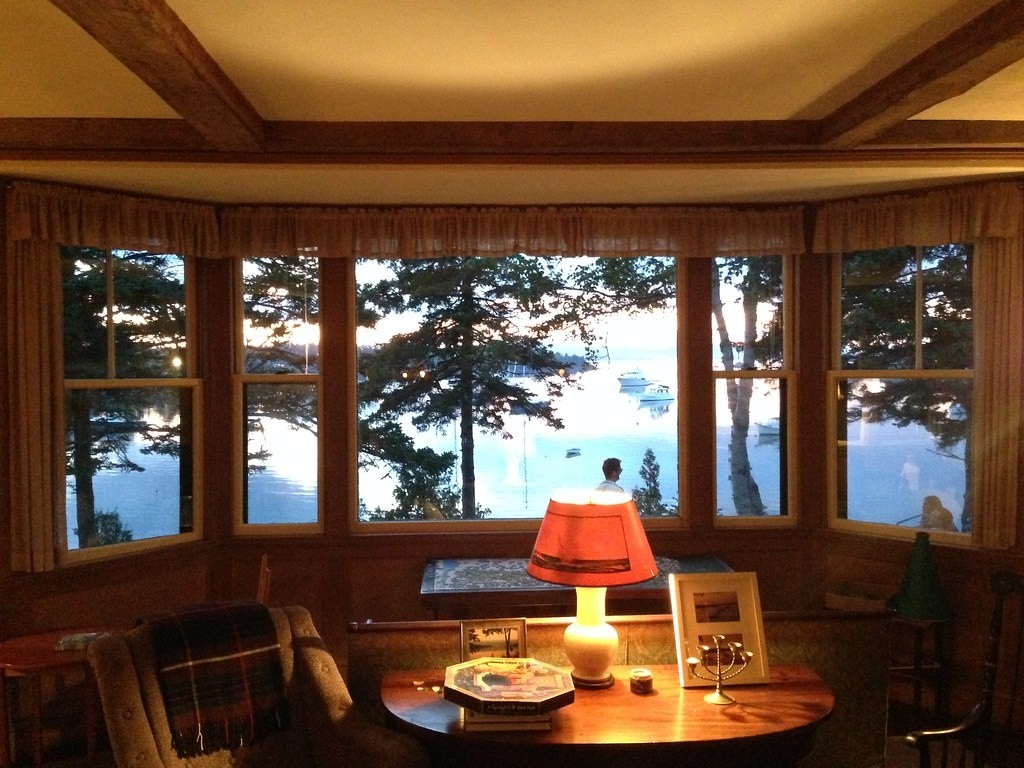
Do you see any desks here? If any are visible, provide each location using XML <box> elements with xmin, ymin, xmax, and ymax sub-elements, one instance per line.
<box><xmin>0</xmin><ymin>625</ymin><xmax>127</xmax><ymax>768</ymax></box>
<box><xmin>419</xmin><ymin>553</ymin><xmax>738</xmax><ymax>620</ymax></box>
<box><xmin>379</xmin><ymin>665</ymin><xmax>837</xmax><ymax>768</ymax></box>
<box><xmin>821</xmin><ymin>605</ymin><xmax>957</xmax><ymax>730</ymax></box>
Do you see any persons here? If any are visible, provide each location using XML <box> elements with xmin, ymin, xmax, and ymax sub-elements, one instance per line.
<box><xmin>595</xmin><ymin>457</ymin><xmax>625</xmax><ymax>494</ymax></box>
<box><xmin>920</xmin><ymin>495</ymin><xmax>958</xmax><ymax>532</ymax></box>
<box><xmin>900</xmin><ymin>456</ymin><xmax>920</xmax><ymax>491</ymax></box>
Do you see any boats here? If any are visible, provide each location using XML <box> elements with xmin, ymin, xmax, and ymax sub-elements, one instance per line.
<box><xmin>754</xmin><ymin>417</ymin><xmax>780</xmax><ymax>436</ymax></box>
<box><xmin>639</xmin><ymin>386</ymin><xmax>675</xmax><ymax>404</ymax></box>
<box><xmin>566</xmin><ymin>447</ymin><xmax>581</xmax><ymax>455</ymax></box>
<box><xmin>618</xmin><ymin>370</ymin><xmax>654</xmax><ymax>387</ymax></box>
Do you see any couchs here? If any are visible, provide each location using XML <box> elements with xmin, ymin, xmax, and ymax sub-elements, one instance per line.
<box><xmin>86</xmin><ymin>605</ymin><xmax>431</xmax><ymax>768</ymax></box>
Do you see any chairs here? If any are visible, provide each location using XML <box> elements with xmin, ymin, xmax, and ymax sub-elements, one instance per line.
<box><xmin>904</xmin><ymin>571</ymin><xmax>1024</xmax><ymax>768</ymax></box>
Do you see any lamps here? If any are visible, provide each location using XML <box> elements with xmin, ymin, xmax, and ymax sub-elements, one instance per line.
<box><xmin>526</xmin><ymin>491</ymin><xmax>658</xmax><ymax>690</ymax></box>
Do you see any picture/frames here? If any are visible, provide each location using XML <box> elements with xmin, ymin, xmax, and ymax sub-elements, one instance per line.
<box><xmin>459</xmin><ymin>618</ymin><xmax>527</xmax><ymax>664</ymax></box>
<box><xmin>668</xmin><ymin>571</ymin><xmax>770</xmax><ymax>689</ymax></box>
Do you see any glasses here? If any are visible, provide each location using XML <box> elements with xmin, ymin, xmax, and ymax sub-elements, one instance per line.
<box><xmin>615</xmin><ymin>468</ymin><xmax>623</xmax><ymax>472</ymax></box>
